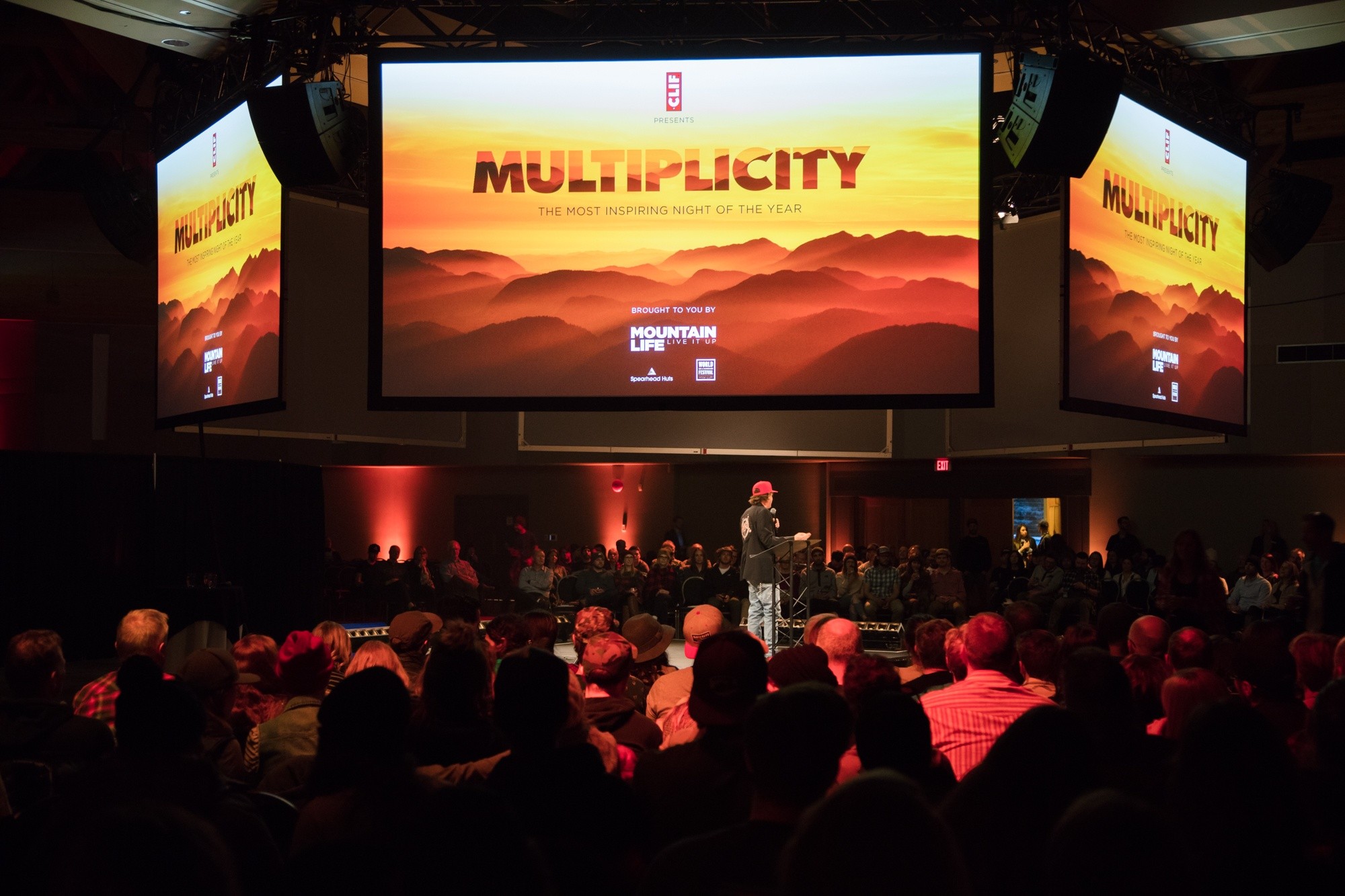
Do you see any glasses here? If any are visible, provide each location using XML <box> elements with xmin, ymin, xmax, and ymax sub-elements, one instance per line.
<box><xmin>879</xmin><ymin>554</ymin><xmax>889</xmax><ymax>557</ymax></box>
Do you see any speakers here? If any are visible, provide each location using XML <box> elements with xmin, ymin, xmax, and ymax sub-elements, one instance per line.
<box><xmin>80</xmin><ymin>163</ymin><xmax>157</xmax><ymax>267</ymax></box>
<box><xmin>243</xmin><ymin>80</ymin><xmax>360</xmax><ymax>191</ymax></box>
<box><xmin>996</xmin><ymin>53</ymin><xmax>1125</xmax><ymax>179</ymax></box>
<box><xmin>1248</xmin><ymin>173</ymin><xmax>1335</xmax><ymax>273</ymax></box>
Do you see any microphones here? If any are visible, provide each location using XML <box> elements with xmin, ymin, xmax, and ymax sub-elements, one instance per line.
<box><xmin>770</xmin><ymin>507</ymin><xmax>778</xmax><ymax>534</ymax></box>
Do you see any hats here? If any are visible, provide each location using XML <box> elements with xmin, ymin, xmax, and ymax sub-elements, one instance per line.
<box><xmin>582</xmin><ymin>631</ymin><xmax>638</xmax><ymax>686</ymax></box>
<box><xmin>752</xmin><ymin>480</ymin><xmax>778</xmax><ymax>496</ymax></box>
<box><xmin>1037</xmin><ymin>520</ymin><xmax>1048</xmax><ymax>530</ymax></box>
<box><xmin>658</xmin><ymin>548</ymin><xmax>671</xmax><ymax>559</ymax></box>
<box><xmin>622</xmin><ymin>612</ymin><xmax>676</xmax><ymax>663</ymax></box>
<box><xmin>1000</xmin><ymin>548</ymin><xmax>1010</xmax><ymax>557</ymax></box>
<box><xmin>589</xmin><ymin>551</ymin><xmax>607</xmax><ymax>564</ymax></box>
<box><xmin>274</xmin><ymin>630</ymin><xmax>334</xmax><ymax>685</ymax></box>
<box><xmin>877</xmin><ymin>546</ymin><xmax>892</xmax><ymax>555</ymax></box>
<box><xmin>688</xmin><ymin>631</ymin><xmax>768</xmax><ymax>726</ymax></box>
<box><xmin>719</xmin><ymin>547</ymin><xmax>733</xmax><ymax>552</ymax></box>
<box><xmin>767</xmin><ymin>643</ymin><xmax>838</xmax><ymax>689</ymax></box>
<box><xmin>179</xmin><ymin>648</ymin><xmax>261</xmax><ymax>691</ymax></box>
<box><xmin>388</xmin><ymin>611</ymin><xmax>443</xmax><ymax>652</ymax></box>
<box><xmin>368</xmin><ymin>544</ymin><xmax>380</xmax><ymax>552</ymax></box>
<box><xmin>574</xmin><ymin>606</ymin><xmax>619</xmax><ymax>643</ymax></box>
<box><xmin>1244</xmin><ymin>555</ymin><xmax>1257</xmax><ymax>567</ymax></box>
<box><xmin>683</xmin><ymin>604</ymin><xmax>725</xmax><ymax>659</ymax></box>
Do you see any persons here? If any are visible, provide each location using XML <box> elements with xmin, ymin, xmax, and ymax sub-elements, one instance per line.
<box><xmin>959</xmin><ymin>510</ymin><xmax>1345</xmax><ymax>636</ymax></box>
<box><xmin>325</xmin><ymin>517</ymin><xmax>965</xmax><ymax>648</ymax></box>
<box><xmin>739</xmin><ymin>481</ymin><xmax>811</xmax><ymax>660</ymax></box>
<box><xmin>0</xmin><ymin>601</ymin><xmax>1345</xmax><ymax>896</ymax></box>
